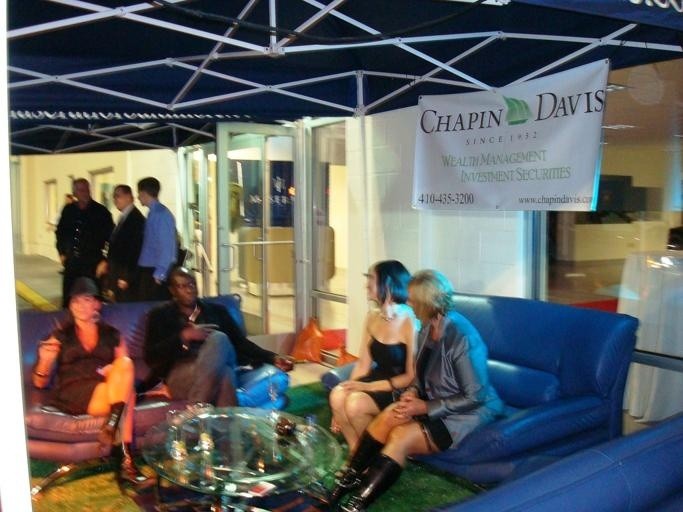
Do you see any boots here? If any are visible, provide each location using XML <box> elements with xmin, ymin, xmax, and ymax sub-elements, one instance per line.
<box><xmin>334</xmin><ymin>453</ymin><xmax>402</xmax><ymax>512</ymax></box>
<box><xmin>94</xmin><ymin>402</ymin><xmax>126</xmax><ymax>446</ymax></box>
<box><xmin>331</xmin><ymin>429</ymin><xmax>384</xmax><ymax>503</ymax></box>
<box><xmin>115</xmin><ymin>441</ymin><xmax>147</xmax><ymax>485</ymax></box>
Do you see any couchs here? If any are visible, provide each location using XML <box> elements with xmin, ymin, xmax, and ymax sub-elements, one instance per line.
<box><xmin>446</xmin><ymin>409</ymin><xmax>683</xmax><ymax>512</ymax></box>
<box><xmin>16</xmin><ymin>290</ymin><xmax>291</xmax><ymax>474</ymax></box>
<box><xmin>318</xmin><ymin>292</ymin><xmax>641</xmax><ymax>490</ymax></box>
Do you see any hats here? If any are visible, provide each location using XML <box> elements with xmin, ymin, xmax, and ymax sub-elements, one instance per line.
<box><xmin>60</xmin><ymin>275</ymin><xmax>113</xmax><ymax>306</ymax></box>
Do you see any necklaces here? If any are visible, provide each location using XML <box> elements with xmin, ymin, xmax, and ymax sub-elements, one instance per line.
<box><xmin>378</xmin><ymin>305</ymin><xmax>403</xmax><ymax>320</ymax></box>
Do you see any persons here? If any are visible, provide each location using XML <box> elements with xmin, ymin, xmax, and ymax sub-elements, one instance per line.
<box><xmin>326</xmin><ymin>268</ymin><xmax>501</xmax><ymax>511</ymax></box>
<box><xmin>139</xmin><ymin>270</ymin><xmax>295</xmax><ymax>410</ymax></box>
<box><xmin>106</xmin><ymin>184</ymin><xmax>148</xmax><ymax>302</ymax></box>
<box><xmin>136</xmin><ymin>177</ymin><xmax>179</xmax><ymax>301</ymax></box>
<box><xmin>33</xmin><ymin>275</ymin><xmax>138</xmax><ymax>482</ymax></box>
<box><xmin>329</xmin><ymin>256</ymin><xmax>422</xmax><ymax>478</ymax></box>
<box><xmin>54</xmin><ymin>178</ymin><xmax>115</xmax><ymax>310</ymax></box>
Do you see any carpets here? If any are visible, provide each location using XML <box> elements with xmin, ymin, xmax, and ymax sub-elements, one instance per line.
<box><xmin>28</xmin><ymin>379</ymin><xmax>487</xmax><ymax>512</ymax></box>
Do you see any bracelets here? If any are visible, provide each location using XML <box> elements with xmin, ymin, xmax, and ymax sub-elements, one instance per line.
<box><xmin>32</xmin><ymin>368</ymin><xmax>51</xmax><ymax>379</ymax></box>
<box><xmin>387</xmin><ymin>377</ymin><xmax>399</xmax><ymax>391</ymax></box>
<box><xmin>406</xmin><ymin>385</ymin><xmax>422</xmax><ymax>396</ymax></box>
